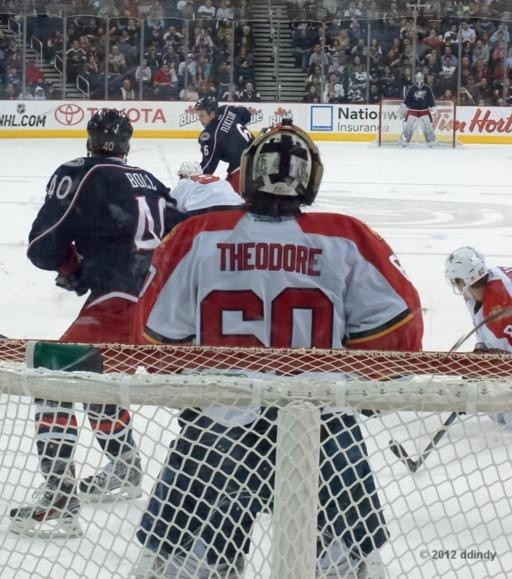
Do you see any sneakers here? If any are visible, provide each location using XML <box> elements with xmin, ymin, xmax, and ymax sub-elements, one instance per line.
<box><xmin>80</xmin><ymin>453</ymin><xmax>142</xmax><ymax>492</ymax></box>
<box><xmin>10</xmin><ymin>488</ymin><xmax>81</xmax><ymax>522</ymax></box>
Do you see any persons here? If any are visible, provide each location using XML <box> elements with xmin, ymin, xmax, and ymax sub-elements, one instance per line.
<box><xmin>144</xmin><ymin>0</ymin><xmax>261</xmax><ymax>101</ymax></box>
<box><xmin>42</xmin><ymin>0</ymin><xmax>143</xmax><ymax>101</ymax></box>
<box><xmin>459</xmin><ymin>1</ymin><xmax>511</xmax><ymax>105</ymax></box>
<box><xmin>171</xmin><ymin>161</ymin><xmax>246</xmax><ymax>211</ymax></box>
<box><xmin>446</xmin><ymin>248</ymin><xmax>511</xmax><ymax>425</ymax></box>
<box><xmin>372</xmin><ymin>70</ymin><xmax>399</xmax><ymax>105</ymax></box>
<box><xmin>288</xmin><ymin>1</ymin><xmax>369</xmax><ymax>104</ymax></box>
<box><xmin>438</xmin><ymin>70</ymin><xmax>456</xmax><ymax>100</ymax></box>
<box><xmin>398</xmin><ymin>71</ymin><xmax>437</xmax><ymax>148</ymax></box>
<box><xmin>0</xmin><ymin>0</ymin><xmax>40</xmax><ymax>100</ymax></box>
<box><xmin>194</xmin><ymin>96</ymin><xmax>256</xmax><ymax>194</ymax></box>
<box><xmin>371</xmin><ymin>1</ymin><xmax>458</xmax><ymax>69</ymax></box>
<box><xmin>8</xmin><ymin>110</ymin><xmax>175</xmax><ymax>541</ymax></box>
<box><xmin>132</xmin><ymin>123</ymin><xmax>424</xmax><ymax>579</ymax></box>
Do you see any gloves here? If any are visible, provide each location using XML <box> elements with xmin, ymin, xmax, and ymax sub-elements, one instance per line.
<box><xmin>56</xmin><ymin>259</ymin><xmax>87</xmax><ymax>296</ymax></box>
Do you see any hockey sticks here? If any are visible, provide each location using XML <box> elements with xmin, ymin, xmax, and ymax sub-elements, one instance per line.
<box><xmin>388</xmin><ymin>411</ymin><xmax>457</xmax><ymax>472</ymax></box>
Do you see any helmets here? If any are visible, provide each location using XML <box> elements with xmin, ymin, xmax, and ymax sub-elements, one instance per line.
<box><xmin>177</xmin><ymin>160</ymin><xmax>203</xmax><ymax>177</ymax></box>
<box><xmin>193</xmin><ymin>95</ymin><xmax>218</xmax><ymax>111</ymax></box>
<box><xmin>415</xmin><ymin>72</ymin><xmax>425</xmax><ymax>88</ymax></box>
<box><xmin>85</xmin><ymin>109</ymin><xmax>134</xmax><ymax>155</ymax></box>
<box><xmin>239</xmin><ymin>118</ymin><xmax>325</xmax><ymax>208</ymax></box>
<box><xmin>444</xmin><ymin>245</ymin><xmax>489</xmax><ymax>295</ymax></box>
